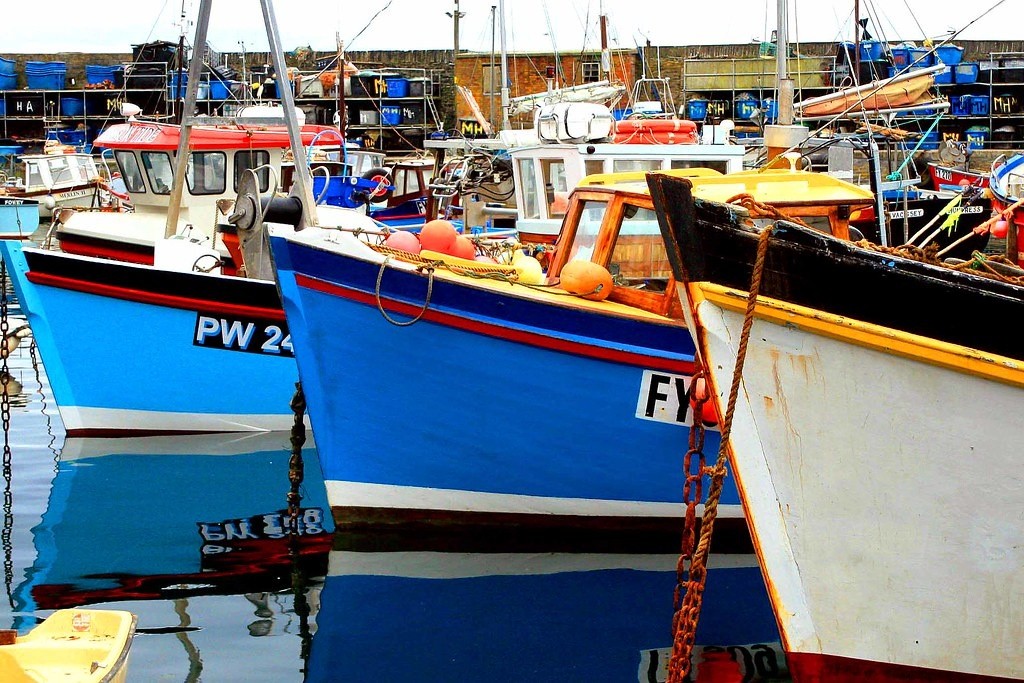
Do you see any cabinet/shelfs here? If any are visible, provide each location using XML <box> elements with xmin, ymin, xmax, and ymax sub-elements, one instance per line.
<box><xmin>169</xmin><ymin>67</ymin><xmax>447</xmax><ymax>156</ymax></box>
<box><xmin>0</xmin><ymin>87</ymin><xmax>169</xmax><ymax>161</ymax></box>
<box><xmin>683</xmin><ymin>58</ymin><xmax>835</xmax><ymax>137</ymax></box>
<box><xmin>837</xmin><ymin>52</ymin><xmax>1024</xmax><ymax>150</ymax></box>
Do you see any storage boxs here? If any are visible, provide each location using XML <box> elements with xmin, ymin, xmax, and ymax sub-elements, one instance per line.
<box><xmin>250</xmin><ymin>53</ymin><xmax>432</xmax><ymax>151</ymax></box>
<box><xmin>168</xmin><ymin>70</ymin><xmax>231</xmax><ymax>102</ymax></box>
<box><xmin>838</xmin><ymin>39</ymin><xmax>1017</xmax><ymax>151</ymax></box>
<box><xmin>0</xmin><ymin>58</ymin><xmax>125</xmax><ymax>172</ymax></box>
<box><xmin>689</xmin><ymin>96</ymin><xmax>778</xmax><ymax>138</ymax></box>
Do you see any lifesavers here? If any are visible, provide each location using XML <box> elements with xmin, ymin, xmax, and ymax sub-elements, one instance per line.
<box><xmin>44</xmin><ymin>145</ymin><xmax>77</xmax><ymax>153</ymax></box>
<box><xmin>609</xmin><ymin>119</ymin><xmax>697</xmax><ymax>144</ymax></box>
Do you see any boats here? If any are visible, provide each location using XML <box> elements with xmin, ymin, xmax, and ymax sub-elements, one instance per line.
<box><xmin>304</xmin><ymin>532</ymin><xmax>792</xmax><ymax>683</ymax></box>
<box><xmin>226</xmin><ymin>1</ymin><xmax>874</xmax><ymax>534</ymax></box>
<box><xmin>0</xmin><ymin>0</ymin><xmax>1023</xmax><ymax>272</ymax></box>
<box><xmin>9</xmin><ymin>429</ymin><xmax>337</xmax><ymax>636</ymax></box>
<box><xmin>0</xmin><ymin>607</ymin><xmax>138</xmax><ymax>682</ymax></box>
<box><xmin>1</xmin><ymin>1</ymin><xmax>317</xmax><ymax>439</ymax></box>
<box><xmin>646</xmin><ymin>171</ymin><xmax>1024</xmax><ymax>683</ymax></box>
